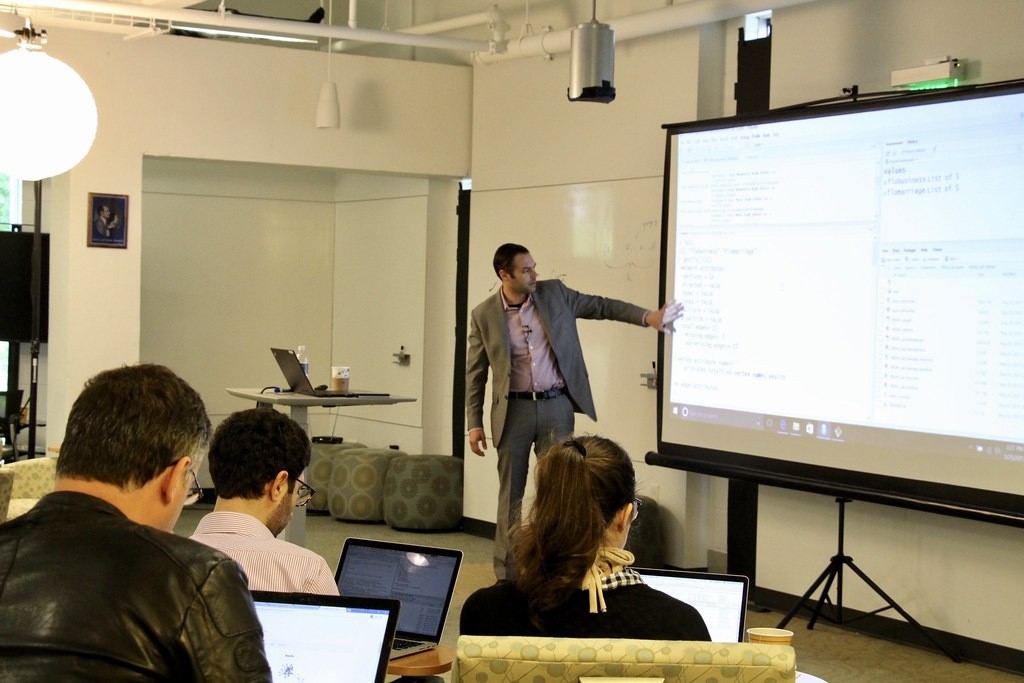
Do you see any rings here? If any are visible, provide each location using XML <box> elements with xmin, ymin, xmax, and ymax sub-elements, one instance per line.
<box><xmin>665</xmin><ymin>308</ymin><xmax>668</xmax><ymax>312</ymax></box>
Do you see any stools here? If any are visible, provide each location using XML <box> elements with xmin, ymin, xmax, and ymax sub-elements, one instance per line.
<box><xmin>328</xmin><ymin>450</ymin><xmax>406</xmax><ymax>522</ymax></box>
<box><xmin>305</xmin><ymin>441</ymin><xmax>367</xmax><ymax>512</ymax></box>
<box><xmin>384</xmin><ymin>454</ymin><xmax>463</xmax><ymax>531</ymax></box>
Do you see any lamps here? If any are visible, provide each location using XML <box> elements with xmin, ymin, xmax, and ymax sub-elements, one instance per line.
<box><xmin>316</xmin><ymin>0</ymin><xmax>341</xmax><ymax>130</ymax></box>
<box><xmin>0</xmin><ymin>22</ymin><xmax>98</xmax><ymax>182</ymax></box>
<box><xmin>567</xmin><ymin>0</ymin><xmax>616</xmax><ymax>104</ymax></box>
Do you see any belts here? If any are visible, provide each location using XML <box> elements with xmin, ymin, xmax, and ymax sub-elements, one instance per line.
<box><xmin>509</xmin><ymin>388</ymin><xmax>566</xmax><ymax>400</ymax></box>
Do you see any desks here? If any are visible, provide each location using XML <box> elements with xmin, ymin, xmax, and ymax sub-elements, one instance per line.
<box><xmin>385</xmin><ymin>646</ymin><xmax>456</xmax><ymax>676</ymax></box>
<box><xmin>227</xmin><ymin>386</ymin><xmax>418</xmax><ymax>547</ymax></box>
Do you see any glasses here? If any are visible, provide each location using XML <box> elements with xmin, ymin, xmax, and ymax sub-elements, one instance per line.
<box><xmin>616</xmin><ymin>496</ymin><xmax>644</xmax><ymax>521</ymax></box>
<box><xmin>287</xmin><ymin>476</ymin><xmax>316</xmax><ymax>509</ymax></box>
<box><xmin>183</xmin><ymin>466</ymin><xmax>204</xmax><ymax>508</ymax></box>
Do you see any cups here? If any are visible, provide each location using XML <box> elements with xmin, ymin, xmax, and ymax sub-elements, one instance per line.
<box><xmin>19</xmin><ymin>406</ymin><xmax>28</xmax><ymax>423</ymax></box>
<box><xmin>747</xmin><ymin>628</ymin><xmax>794</xmax><ymax>645</ymax></box>
<box><xmin>332</xmin><ymin>367</ymin><xmax>350</xmax><ymax>392</ymax></box>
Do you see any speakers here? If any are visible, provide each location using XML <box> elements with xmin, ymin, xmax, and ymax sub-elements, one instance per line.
<box><xmin>0</xmin><ymin>231</ymin><xmax>51</xmax><ymax>342</ymax></box>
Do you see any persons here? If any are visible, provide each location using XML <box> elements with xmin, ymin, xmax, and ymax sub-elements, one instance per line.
<box><xmin>93</xmin><ymin>204</ymin><xmax>118</xmax><ymax>244</ymax></box>
<box><xmin>465</xmin><ymin>243</ymin><xmax>684</xmax><ymax>580</ymax></box>
<box><xmin>0</xmin><ymin>364</ymin><xmax>272</xmax><ymax>683</ymax></box>
<box><xmin>187</xmin><ymin>408</ymin><xmax>340</xmax><ymax>596</ymax></box>
<box><xmin>459</xmin><ymin>437</ymin><xmax>711</xmax><ymax>641</ymax></box>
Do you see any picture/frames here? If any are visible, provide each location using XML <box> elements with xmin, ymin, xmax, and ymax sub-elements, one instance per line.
<box><xmin>87</xmin><ymin>191</ymin><xmax>130</xmax><ymax>248</ymax></box>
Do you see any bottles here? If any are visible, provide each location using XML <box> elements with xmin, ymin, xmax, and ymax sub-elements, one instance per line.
<box><xmin>296</xmin><ymin>345</ymin><xmax>308</xmax><ymax>379</ymax></box>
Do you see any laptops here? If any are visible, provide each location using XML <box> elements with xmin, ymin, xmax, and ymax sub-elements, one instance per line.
<box><xmin>271</xmin><ymin>348</ymin><xmax>390</xmax><ymax>398</ymax></box>
<box><xmin>247</xmin><ymin>590</ymin><xmax>401</xmax><ymax>683</ymax></box>
<box><xmin>626</xmin><ymin>567</ymin><xmax>750</xmax><ymax>642</ymax></box>
<box><xmin>335</xmin><ymin>537</ymin><xmax>464</xmax><ymax>661</ymax></box>
